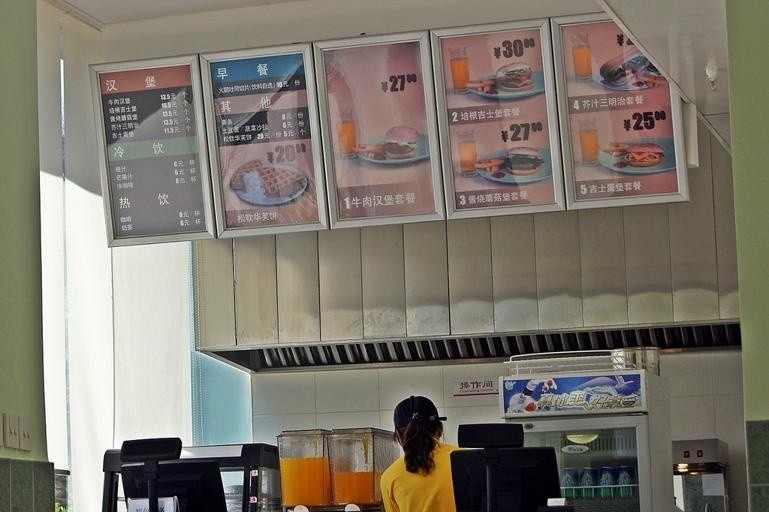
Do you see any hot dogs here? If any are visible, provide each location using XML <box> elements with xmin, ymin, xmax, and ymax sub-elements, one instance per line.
<box><xmin>600</xmin><ymin>46</ymin><xmax>651</xmax><ymax>87</ymax></box>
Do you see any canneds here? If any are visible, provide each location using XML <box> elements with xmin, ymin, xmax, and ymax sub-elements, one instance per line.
<box><xmin>600</xmin><ymin>465</ymin><xmax>617</xmax><ymax>500</ymax></box>
<box><xmin>617</xmin><ymin>466</ymin><xmax>635</xmax><ymax>498</ymax></box>
<box><xmin>561</xmin><ymin>467</ymin><xmax>578</xmax><ymax>500</ymax></box>
<box><xmin>580</xmin><ymin>466</ymin><xmax>597</xmax><ymax>499</ymax></box>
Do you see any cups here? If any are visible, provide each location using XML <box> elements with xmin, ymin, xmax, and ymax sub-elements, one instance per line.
<box><xmin>336</xmin><ymin>110</ymin><xmax>357</xmax><ymax>157</ymax></box>
<box><xmin>448</xmin><ymin>46</ymin><xmax>468</xmax><ymax>94</ymax></box>
<box><xmin>578</xmin><ymin>117</ymin><xmax>599</xmax><ymax>167</ymax></box>
<box><xmin>458</xmin><ymin>130</ymin><xmax>477</xmax><ymax>179</ymax></box>
<box><xmin>571</xmin><ymin>33</ymin><xmax>593</xmax><ymax>81</ymax></box>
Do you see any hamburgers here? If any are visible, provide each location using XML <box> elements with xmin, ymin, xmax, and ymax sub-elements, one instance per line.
<box><xmin>505</xmin><ymin>147</ymin><xmax>545</xmax><ymax>175</ymax></box>
<box><xmin>495</xmin><ymin>62</ymin><xmax>534</xmax><ymax>92</ymax></box>
<box><xmin>625</xmin><ymin>143</ymin><xmax>665</xmax><ymax>166</ymax></box>
<box><xmin>383</xmin><ymin>126</ymin><xmax>419</xmax><ymax>159</ymax></box>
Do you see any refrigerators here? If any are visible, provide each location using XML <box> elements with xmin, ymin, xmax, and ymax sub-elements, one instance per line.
<box><xmin>501</xmin><ymin>376</ymin><xmax>650</xmax><ymax>512</ymax></box>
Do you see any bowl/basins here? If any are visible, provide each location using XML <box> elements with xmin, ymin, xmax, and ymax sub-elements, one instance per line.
<box><xmin>613</xmin><ymin>347</ymin><xmax>661</xmax><ymax>375</ymax></box>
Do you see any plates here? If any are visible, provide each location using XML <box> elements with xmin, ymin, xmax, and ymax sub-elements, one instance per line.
<box><xmin>594</xmin><ymin>70</ymin><xmax>649</xmax><ymax>90</ymax></box>
<box><xmin>358</xmin><ymin>135</ymin><xmax>428</xmax><ymax>165</ymax></box>
<box><xmin>470</xmin><ymin>73</ymin><xmax>545</xmax><ymax>100</ymax></box>
<box><xmin>599</xmin><ymin>138</ymin><xmax>676</xmax><ymax>174</ymax></box>
<box><xmin>477</xmin><ymin>148</ymin><xmax>552</xmax><ymax>185</ymax></box>
<box><xmin>232</xmin><ymin>164</ymin><xmax>307</xmax><ymax>206</ymax></box>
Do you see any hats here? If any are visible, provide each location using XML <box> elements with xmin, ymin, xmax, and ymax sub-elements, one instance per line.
<box><xmin>394</xmin><ymin>396</ymin><xmax>447</xmax><ymax>428</ymax></box>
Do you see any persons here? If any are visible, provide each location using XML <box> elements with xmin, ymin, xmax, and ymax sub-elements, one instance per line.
<box><xmin>379</xmin><ymin>395</ymin><xmax>465</xmax><ymax>512</ymax></box>
